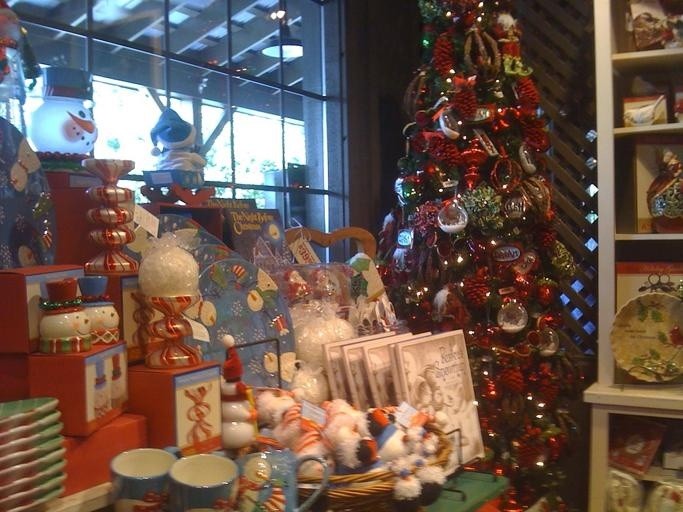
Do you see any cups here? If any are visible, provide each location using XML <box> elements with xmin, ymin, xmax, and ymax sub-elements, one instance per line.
<box><xmin>110</xmin><ymin>448</ymin><xmax>331</xmax><ymax>512</ymax></box>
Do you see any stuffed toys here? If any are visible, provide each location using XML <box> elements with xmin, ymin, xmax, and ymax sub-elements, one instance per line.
<box><xmin>253</xmin><ymin>385</ymin><xmax>452</xmax><ymax>504</ymax></box>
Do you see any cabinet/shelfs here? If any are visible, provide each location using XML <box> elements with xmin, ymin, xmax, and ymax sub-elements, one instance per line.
<box><xmin>581</xmin><ymin>1</ymin><xmax>683</xmax><ymax>511</ymax></box>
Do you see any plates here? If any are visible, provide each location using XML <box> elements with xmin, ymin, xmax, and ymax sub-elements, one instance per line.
<box><xmin>0</xmin><ymin>396</ymin><xmax>68</xmax><ymax>512</ymax></box>
<box><xmin>151</xmin><ymin>213</ymin><xmax>297</xmax><ymax>392</ymax></box>
<box><xmin>0</xmin><ymin>118</ymin><xmax>57</xmax><ymax>270</ymax></box>
<box><xmin>608</xmin><ymin>293</ymin><xmax>683</xmax><ymax>384</ymax></box>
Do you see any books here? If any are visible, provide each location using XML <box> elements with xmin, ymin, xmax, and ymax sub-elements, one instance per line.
<box><xmin>222</xmin><ymin>206</ymin><xmax>293</xmax><ymax>263</ymax></box>
<box><xmin>208</xmin><ymin>198</ymin><xmax>259</xmax><ymax>244</ymax></box>
<box><xmin>321</xmin><ymin>327</ymin><xmax>486</xmax><ymax>479</ymax></box>
<box><xmin>608</xmin><ymin>414</ymin><xmax>668</xmax><ymax>477</ymax></box>
